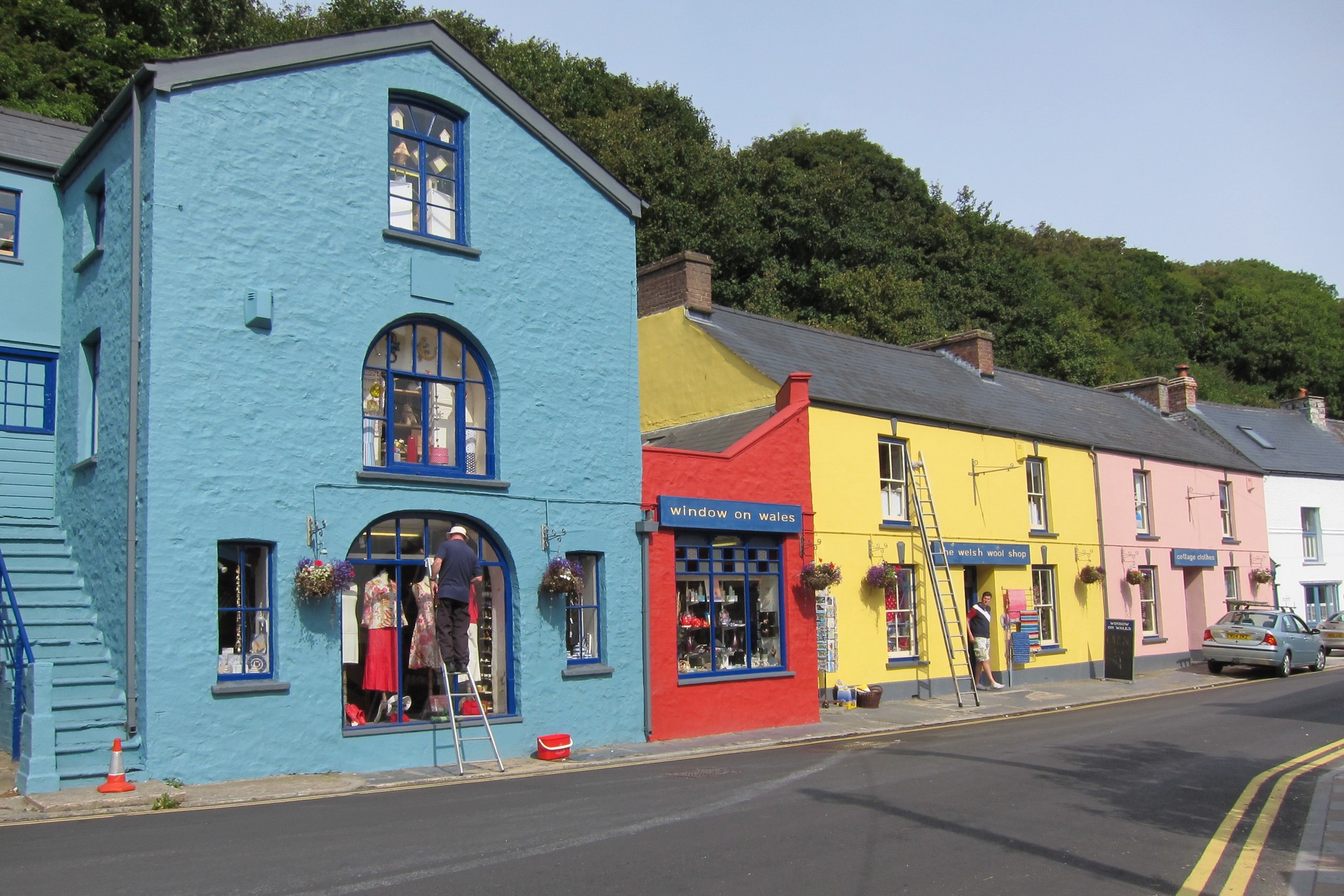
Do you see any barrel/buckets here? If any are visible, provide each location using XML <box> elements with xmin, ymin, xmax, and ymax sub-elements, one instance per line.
<box><xmin>537</xmin><ymin>733</ymin><xmax>570</xmax><ymax>759</ymax></box>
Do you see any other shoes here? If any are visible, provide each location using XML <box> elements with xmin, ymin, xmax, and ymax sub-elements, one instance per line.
<box><xmin>971</xmin><ymin>683</ymin><xmax>986</xmax><ymax>690</ymax></box>
<box><xmin>990</xmin><ymin>682</ymin><xmax>1004</xmax><ymax>689</ymax></box>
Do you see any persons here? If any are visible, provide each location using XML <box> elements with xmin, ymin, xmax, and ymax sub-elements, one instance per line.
<box><xmin>430</xmin><ymin>526</ymin><xmax>482</xmax><ymax>673</ymax></box>
<box><xmin>458</xmin><ymin>584</ymin><xmax>481</xmax><ymax>685</ymax></box>
<box><xmin>966</xmin><ymin>592</ymin><xmax>1005</xmax><ymax>691</ymax></box>
<box><xmin>407</xmin><ymin>574</ymin><xmax>441</xmax><ymax>668</ymax></box>
<box><xmin>360</xmin><ymin>571</ymin><xmax>409</xmax><ymax>693</ymax></box>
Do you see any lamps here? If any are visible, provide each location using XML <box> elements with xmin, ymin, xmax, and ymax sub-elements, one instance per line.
<box><xmin>218</xmin><ymin>561</ymin><xmax>227</xmax><ymax>573</ymax></box>
<box><xmin>376</xmin><ymin>345</ymin><xmax>381</xmax><ymax>356</ymax></box>
<box><xmin>457</xmin><ymin>350</ymin><xmax>462</xmax><ymax>367</ymax></box>
<box><xmin>358</xmin><ymin>535</ymin><xmax>366</xmax><ymax>548</ymax></box>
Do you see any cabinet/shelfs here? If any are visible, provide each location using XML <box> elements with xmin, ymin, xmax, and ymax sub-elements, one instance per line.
<box><xmin>685</xmin><ymin>581</ymin><xmax>745</xmax><ymax>666</ymax></box>
<box><xmin>760</xmin><ymin>611</ymin><xmax>779</xmax><ymax>637</ymax></box>
<box><xmin>390</xmin><ymin>166</ymin><xmax>420</xmax><ymax>231</ymax></box>
<box><xmin>478</xmin><ymin>581</ymin><xmax>493</xmax><ymax>713</ymax></box>
<box><xmin>381</xmin><ymin>386</ymin><xmax>431</xmax><ymax>429</ymax></box>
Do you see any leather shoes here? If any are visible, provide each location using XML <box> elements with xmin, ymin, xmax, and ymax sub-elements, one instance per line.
<box><xmin>447</xmin><ymin>663</ymin><xmax>456</xmax><ymax>672</ymax></box>
<box><xmin>454</xmin><ymin>663</ymin><xmax>467</xmax><ymax>672</ymax></box>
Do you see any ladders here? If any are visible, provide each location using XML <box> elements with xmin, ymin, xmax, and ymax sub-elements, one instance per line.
<box><xmin>906</xmin><ymin>450</ymin><xmax>981</xmax><ymax>708</ymax></box>
<box><xmin>425</xmin><ymin>557</ymin><xmax>505</xmax><ymax>776</ymax></box>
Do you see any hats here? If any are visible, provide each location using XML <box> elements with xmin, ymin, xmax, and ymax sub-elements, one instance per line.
<box><xmin>446</xmin><ymin>525</ymin><xmax>469</xmax><ymax>541</ymax></box>
<box><xmin>386</xmin><ymin>694</ymin><xmax>412</xmax><ymax>716</ymax></box>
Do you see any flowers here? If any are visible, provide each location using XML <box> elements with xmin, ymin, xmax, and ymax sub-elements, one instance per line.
<box><xmin>1079</xmin><ymin>564</ymin><xmax>1106</xmax><ymax>592</ymax></box>
<box><xmin>293</xmin><ymin>551</ymin><xmax>354</xmax><ymax>627</ymax></box>
<box><xmin>799</xmin><ymin>557</ymin><xmax>844</xmax><ymax>608</ymax></box>
<box><xmin>1125</xmin><ymin>569</ymin><xmax>1152</xmax><ymax>587</ymax></box>
<box><xmin>1253</xmin><ymin>568</ymin><xmax>1276</xmax><ymax>590</ymax></box>
<box><xmin>864</xmin><ymin>561</ymin><xmax>903</xmax><ymax>597</ymax></box>
<box><xmin>540</xmin><ymin>556</ymin><xmax>584</xmax><ymax>639</ymax></box>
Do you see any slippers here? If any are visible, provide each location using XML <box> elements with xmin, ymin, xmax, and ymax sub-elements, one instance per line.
<box><xmin>350</xmin><ymin>704</ymin><xmax>366</xmax><ymax>725</ymax></box>
<box><xmin>345</xmin><ymin>704</ymin><xmax>361</xmax><ymax>726</ymax></box>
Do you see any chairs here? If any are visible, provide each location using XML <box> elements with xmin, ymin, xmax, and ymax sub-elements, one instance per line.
<box><xmin>1262</xmin><ymin>620</ymin><xmax>1273</xmax><ymax>627</ymax></box>
<box><xmin>1243</xmin><ymin>618</ymin><xmax>1254</xmax><ymax>625</ymax></box>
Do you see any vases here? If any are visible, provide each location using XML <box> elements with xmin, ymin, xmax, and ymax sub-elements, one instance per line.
<box><xmin>879</xmin><ymin>582</ymin><xmax>892</xmax><ymax>589</ymax></box>
<box><xmin>1130</xmin><ymin>579</ymin><xmax>1141</xmax><ymax>585</ymax></box>
<box><xmin>810</xmin><ymin>583</ymin><xmax>828</xmax><ymax>591</ymax></box>
<box><xmin>1082</xmin><ymin>577</ymin><xmax>1096</xmax><ymax>584</ymax></box>
<box><xmin>1255</xmin><ymin>576</ymin><xmax>1269</xmax><ymax>584</ymax></box>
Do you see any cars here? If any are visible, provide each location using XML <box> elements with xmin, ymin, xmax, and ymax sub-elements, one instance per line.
<box><xmin>1316</xmin><ymin>610</ymin><xmax>1344</xmax><ymax>655</ymax></box>
<box><xmin>1202</xmin><ymin>597</ymin><xmax>1326</xmax><ymax>677</ymax></box>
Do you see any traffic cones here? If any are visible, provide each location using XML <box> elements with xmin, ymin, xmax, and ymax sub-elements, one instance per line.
<box><xmin>97</xmin><ymin>738</ymin><xmax>136</xmax><ymax>793</ymax></box>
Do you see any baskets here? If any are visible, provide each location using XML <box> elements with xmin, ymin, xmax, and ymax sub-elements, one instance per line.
<box><xmin>857</xmin><ymin>685</ymin><xmax>883</xmax><ymax>708</ymax></box>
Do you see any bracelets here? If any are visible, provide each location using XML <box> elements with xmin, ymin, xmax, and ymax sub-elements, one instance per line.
<box><xmin>430</xmin><ymin>578</ymin><xmax>436</xmax><ymax>581</ymax></box>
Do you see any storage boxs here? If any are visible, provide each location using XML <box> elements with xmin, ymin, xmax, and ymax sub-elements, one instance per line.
<box><xmin>836</xmin><ymin>684</ymin><xmax>857</xmax><ymax>710</ymax></box>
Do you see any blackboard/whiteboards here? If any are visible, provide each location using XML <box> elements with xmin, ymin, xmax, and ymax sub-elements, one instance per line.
<box><xmin>1104</xmin><ymin>618</ymin><xmax>1135</xmax><ymax>681</ymax></box>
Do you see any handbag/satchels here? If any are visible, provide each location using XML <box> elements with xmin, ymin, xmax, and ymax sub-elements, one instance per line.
<box><xmin>462</xmin><ymin>700</ymin><xmax>488</xmax><ymax>716</ymax></box>
